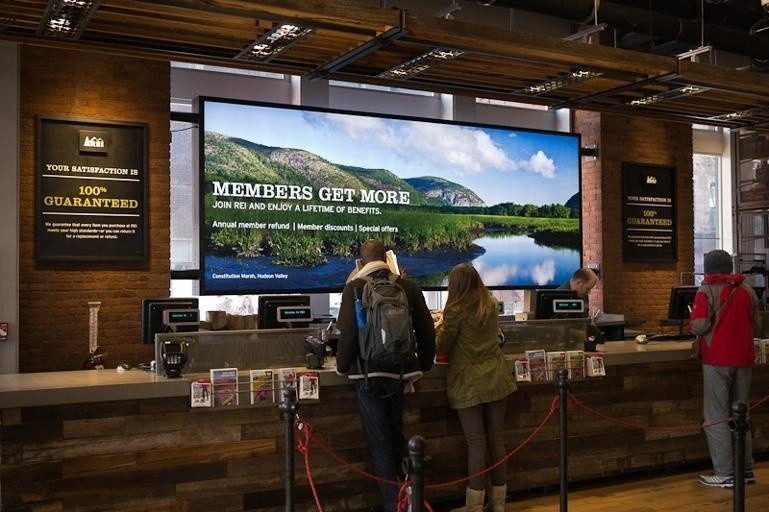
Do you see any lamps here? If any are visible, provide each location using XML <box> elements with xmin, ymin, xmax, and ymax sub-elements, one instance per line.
<box><xmin>562</xmin><ymin>0</ymin><xmax>608</xmax><ymax>40</ymax></box>
<box><xmin>678</xmin><ymin>0</ymin><xmax>712</xmax><ymax>60</ymax></box>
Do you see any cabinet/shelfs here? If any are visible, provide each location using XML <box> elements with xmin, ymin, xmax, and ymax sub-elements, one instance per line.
<box><xmin>730</xmin><ymin>129</ymin><xmax>768</xmax><ymax>339</ymax></box>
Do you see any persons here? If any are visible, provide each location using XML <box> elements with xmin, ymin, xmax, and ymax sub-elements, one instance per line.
<box><xmin>556</xmin><ymin>268</ymin><xmax>597</xmax><ymax>318</ymax></box>
<box><xmin>436</xmin><ymin>263</ymin><xmax>518</xmax><ymax>512</ymax></box>
<box><xmin>688</xmin><ymin>249</ymin><xmax>759</xmax><ymax>488</ymax></box>
<box><xmin>337</xmin><ymin>241</ymin><xmax>436</xmax><ymax>512</ymax></box>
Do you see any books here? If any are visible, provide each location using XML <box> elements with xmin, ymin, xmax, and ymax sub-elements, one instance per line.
<box><xmin>191</xmin><ymin>368</ymin><xmax>320</xmax><ymax>408</ymax></box>
<box><xmin>514</xmin><ymin>350</ymin><xmax>607</xmax><ymax>384</ymax></box>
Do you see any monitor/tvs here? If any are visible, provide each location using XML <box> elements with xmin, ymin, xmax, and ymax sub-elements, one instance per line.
<box><xmin>668</xmin><ymin>285</ymin><xmax>699</xmax><ymax>320</ymax></box>
<box><xmin>141</xmin><ymin>298</ymin><xmax>200</xmax><ymax>345</ymax></box>
<box><xmin>535</xmin><ymin>289</ymin><xmax>586</xmax><ymax>320</ymax></box>
<box><xmin>257</xmin><ymin>296</ymin><xmax>313</xmax><ymax>329</ymax></box>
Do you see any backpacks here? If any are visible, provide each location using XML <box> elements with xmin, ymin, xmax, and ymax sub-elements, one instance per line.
<box><xmin>358</xmin><ymin>273</ymin><xmax>420</xmax><ymax>362</ymax></box>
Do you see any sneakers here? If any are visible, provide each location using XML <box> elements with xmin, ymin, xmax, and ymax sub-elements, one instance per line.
<box><xmin>697</xmin><ymin>468</ymin><xmax>755</xmax><ymax>489</ymax></box>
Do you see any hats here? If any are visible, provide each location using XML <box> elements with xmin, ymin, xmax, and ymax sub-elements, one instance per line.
<box><xmin>704</xmin><ymin>250</ymin><xmax>733</xmax><ymax>273</ymax></box>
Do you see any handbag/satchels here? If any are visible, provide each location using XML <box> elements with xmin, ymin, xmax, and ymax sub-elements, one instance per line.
<box><xmin>689</xmin><ymin>341</ymin><xmax>701</xmax><ymax>361</ymax></box>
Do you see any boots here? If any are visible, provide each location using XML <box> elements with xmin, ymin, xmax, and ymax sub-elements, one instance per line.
<box><xmin>484</xmin><ymin>484</ymin><xmax>507</xmax><ymax>512</ymax></box>
<box><xmin>449</xmin><ymin>485</ymin><xmax>486</xmax><ymax>512</ymax></box>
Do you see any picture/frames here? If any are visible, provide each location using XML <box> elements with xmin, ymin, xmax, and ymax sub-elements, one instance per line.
<box><xmin>622</xmin><ymin>160</ymin><xmax>678</xmax><ymax>263</ymax></box>
<box><xmin>34</xmin><ymin>113</ymin><xmax>149</xmax><ymax>264</ymax></box>
